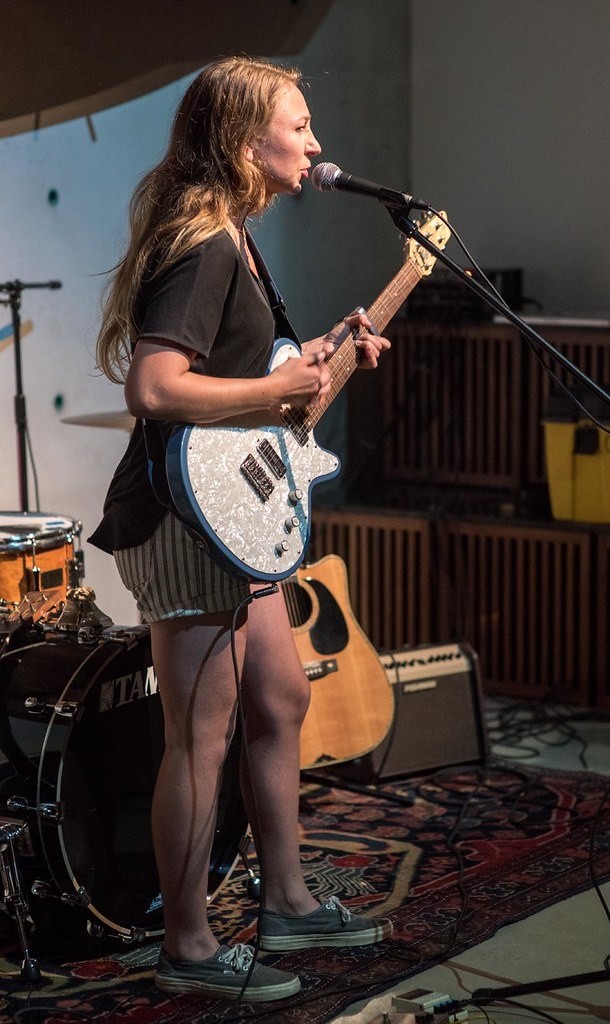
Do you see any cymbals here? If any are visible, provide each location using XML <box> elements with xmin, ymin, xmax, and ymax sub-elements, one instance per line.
<box><xmin>59</xmin><ymin>408</ymin><xmax>141</xmax><ymax>432</ymax></box>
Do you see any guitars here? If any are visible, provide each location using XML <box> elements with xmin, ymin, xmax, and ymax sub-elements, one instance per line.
<box><xmin>163</xmin><ymin>206</ymin><xmax>454</xmax><ymax>587</ymax></box>
<box><xmin>278</xmin><ymin>553</ymin><xmax>399</xmax><ymax>776</ymax></box>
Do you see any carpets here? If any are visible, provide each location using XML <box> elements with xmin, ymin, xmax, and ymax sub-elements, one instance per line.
<box><xmin>0</xmin><ymin>755</ymin><xmax>610</xmax><ymax>1024</ymax></box>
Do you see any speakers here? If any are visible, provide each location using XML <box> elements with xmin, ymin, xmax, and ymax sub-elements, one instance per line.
<box><xmin>339</xmin><ymin>641</ymin><xmax>490</xmax><ymax>782</ymax></box>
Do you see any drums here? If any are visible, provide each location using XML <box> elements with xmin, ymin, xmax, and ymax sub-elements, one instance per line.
<box><xmin>0</xmin><ymin>508</ymin><xmax>82</xmax><ymax>636</ymax></box>
<box><xmin>4</xmin><ymin>625</ymin><xmax>251</xmax><ymax>943</ymax></box>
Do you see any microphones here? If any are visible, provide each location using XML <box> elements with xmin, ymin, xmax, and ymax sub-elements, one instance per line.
<box><xmin>312</xmin><ymin>163</ymin><xmax>431</xmax><ymax>211</ymax></box>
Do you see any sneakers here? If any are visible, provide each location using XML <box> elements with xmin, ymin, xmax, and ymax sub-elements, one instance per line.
<box><xmin>155</xmin><ymin>941</ymin><xmax>301</xmax><ymax>1002</ymax></box>
<box><xmin>257</xmin><ymin>896</ymin><xmax>394</xmax><ymax>950</ymax></box>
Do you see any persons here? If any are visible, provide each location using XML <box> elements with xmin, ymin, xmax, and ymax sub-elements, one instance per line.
<box><xmin>87</xmin><ymin>57</ymin><xmax>393</xmax><ymax>1000</ymax></box>
